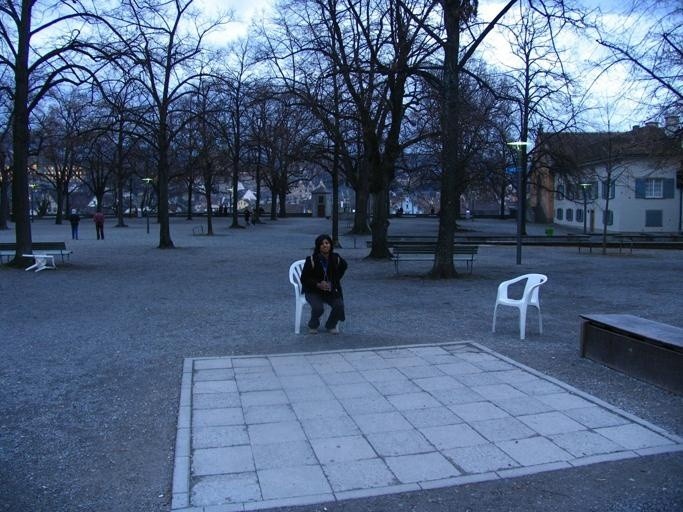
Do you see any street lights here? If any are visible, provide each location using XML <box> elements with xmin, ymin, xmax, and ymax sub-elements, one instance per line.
<box><xmin>138</xmin><ymin>177</ymin><xmax>152</xmax><ymax>233</ymax></box>
<box><xmin>579</xmin><ymin>182</ymin><xmax>592</xmax><ymax>233</ymax></box>
<box><xmin>505</xmin><ymin>140</ymin><xmax>534</xmax><ymax>266</ymax></box>
<box><xmin>28</xmin><ymin>183</ymin><xmax>38</xmax><ymax>223</ymax></box>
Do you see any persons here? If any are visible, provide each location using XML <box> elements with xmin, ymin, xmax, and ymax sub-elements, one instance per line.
<box><xmin>70</xmin><ymin>208</ymin><xmax>80</xmax><ymax>240</ymax></box>
<box><xmin>466</xmin><ymin>208</ymin><xmax>471</xmax><ymax>216</ymax></box>
<box><xmin>431</xmin><ymin>207</ymin><xmax>435</xmax><ymax>215</ymax></box>
<box><xmin>219</xmin><ymin>205</ymin><xmax>222</xmax><ymax>217</ymax></box>
<box><xmin>300</xmin><ymin>234</ymin><xmax>348</xmax><ymax>335</ymax></box>
<box><xmin>224</xmin><ymin>204</ymin><xmax>228</xmax><ymax>215</ymax></box>
<box><xmin>396</xmin><ymin>207</ymin><xmax>403</xmax><ymax>217</ymax></box>
<box><xmin>93</xmin><ymin>207</ymin><xmax>105</xmax><ymax>240</ymax></box>
<box><xmin>243</xmin><ymin>206</ymin><xmax>250</xmax><ymax>225</ymax></box>
<box><xmin>252</xmin><ymin>208</ymin><xmax>258</xmax><ymax>226</ymax></box>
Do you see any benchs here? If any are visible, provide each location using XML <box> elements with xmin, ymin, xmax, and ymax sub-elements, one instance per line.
<box><xmin>389</xmin><ymin>243</ymin><xmax>479</xmax><ymax>276</ymax></box>
<box><xmin>0</xmin><ymin>241</ymin><xmax>73</xmax><ymax>265</ymax></box>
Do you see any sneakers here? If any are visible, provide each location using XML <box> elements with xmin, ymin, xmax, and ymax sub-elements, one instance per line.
<box><xmin>327</xmin><ymin>327</ymin><xmax>337</xmax><ymax>334</ymax></box>
<box><xmin>309</xmin><ymin>328</ymin><xmax>318</xmax><ymax>334</ymax></box>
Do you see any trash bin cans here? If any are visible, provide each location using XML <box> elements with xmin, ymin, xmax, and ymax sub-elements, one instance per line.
<box><xmin>545</xmin><ymin>227</ymin><xmax>553</xmax><ymax>236</ymax></box>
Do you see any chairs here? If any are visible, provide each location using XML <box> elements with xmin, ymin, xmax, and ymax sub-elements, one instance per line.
<box><xmin>288</xmin><ymin>259</ymin><xmax>343</xmax><ymax>335</ymax></box>
<box><xmin>491</xmin><ymin>273</ymin><xmax>548</xmax><ymax>340</ymax></box>
